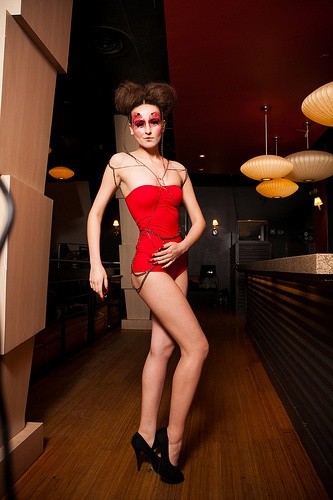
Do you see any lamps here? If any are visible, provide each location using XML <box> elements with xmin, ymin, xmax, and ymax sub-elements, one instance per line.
<box><xmin>283</xmin><ymin>122</ymin><xmax>333</xmax><ymax>183</ymax></box>
<box><xmin>313</xmin><ymin>197</ymin><xmax>323</xmax><ymax>213</ymax></box>
<box><xmin>255</xmin><ymin>135</ymin><xmax>300</xmax><ymax>199</ymax></box>
<box><xmin>49</xmin><ymin>167</ymin><xmax>76</xmax><ymax>180</ymax></box>
<box><xmin>212</xmin><ymin>220</ymin><xmax>218</xmax><ymax>236</ymax></box>
<box><xmin>112</xmin><ymin>220</ymin><xmax>120</xmax><ymax>236</ymax></box>
<box><xmin>240</xmin><ymin>102</ymin><xmax>293</xmax><ymax>180</ymax></box>
<box><xmin>301</xmin><ymin>81</ymin><xmax>333</xmax><ymax>128</ymax></box>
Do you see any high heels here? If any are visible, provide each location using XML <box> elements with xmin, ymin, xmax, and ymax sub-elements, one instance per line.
<box><xmin>151</xmin><ymin>427</ymin><xmax>185</xmax><ymax>484</ymax></box>
<box><xmin>131</xmin><ymin>432</ymin><xmax>157</xmax><ymax>471</ymax></box>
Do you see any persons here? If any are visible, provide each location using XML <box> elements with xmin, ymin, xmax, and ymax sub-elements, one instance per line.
<box><xmin>87</xmin><ymin>81</ymin><xmax>209</xmax><ymax>485</ymax></box>
